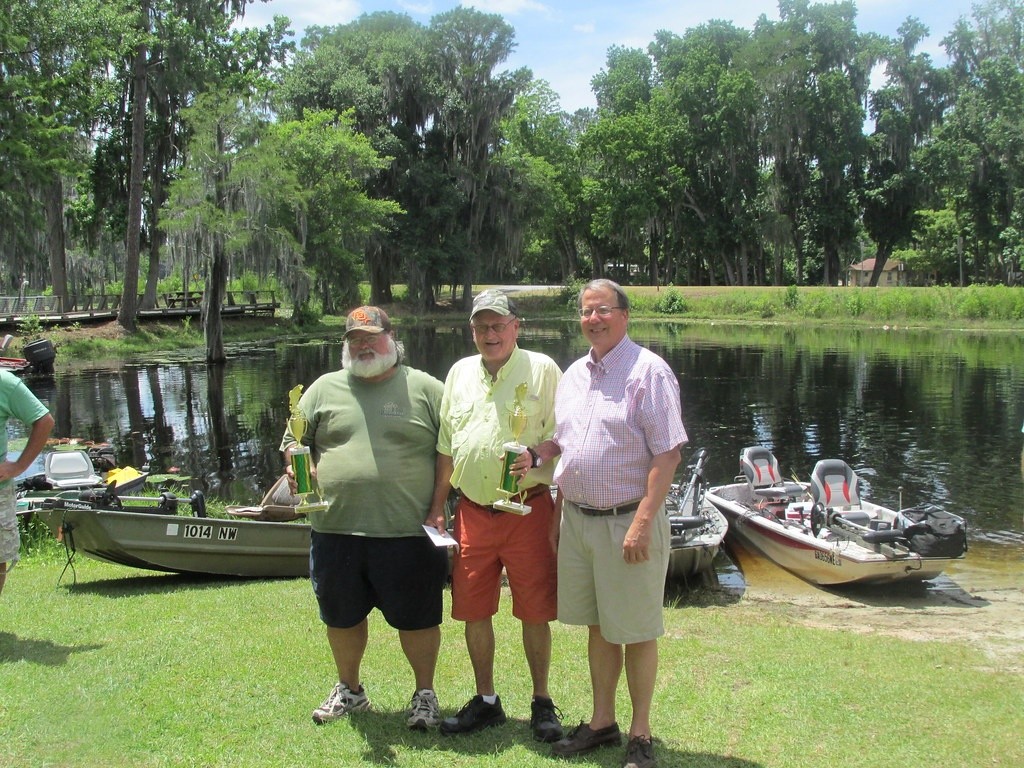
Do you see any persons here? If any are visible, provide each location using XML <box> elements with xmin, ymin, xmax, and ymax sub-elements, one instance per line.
<box><xmin>279</xmin><ymin>306</ymin><xmax>454</xmax><ymax>731</ymax></box>
<box><xmin>0</xmin><ymin>367</ymin><xmax>55</xmax><ymax>596</ymax></box>
<box><xmin>546</xmin><ymin>278</ymin><xmax>689</xmax><ymax>768</ymax></box>
<box><xmin>425</xmin><ymin>289</ymin><xmax>566</xmax><ymax>741</ymax></box>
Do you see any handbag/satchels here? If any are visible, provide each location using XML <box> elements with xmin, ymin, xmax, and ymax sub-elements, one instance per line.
<box><xmin>894</xmin><ymin>503</ymin><xmax>967</xmax><ymax>557</ymax></box>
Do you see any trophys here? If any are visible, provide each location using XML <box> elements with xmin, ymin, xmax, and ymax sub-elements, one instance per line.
<box><xmin>285</xmin><ymin>383</ymin><xmax>328</xmax><ymax>514</ymax></box>
<box><xmin>493</xmin><ymin>381</ymin><xmax>533</xmax><ymax>516</ymax></box>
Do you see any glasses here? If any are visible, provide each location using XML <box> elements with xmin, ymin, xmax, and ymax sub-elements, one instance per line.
<box><xmin>346</xmin><ymin>333</ymin><xmax>386</xmax><ymax>347</ymax></box>
<box><xmin>470</xmin><ymin>318</ymin><xmax>516</xmax><ymax>331</ymax></box>
<box><xmin>579</xmin><ymin>306</ymin><xmax>623</xmax><ymax>317</ymax></box>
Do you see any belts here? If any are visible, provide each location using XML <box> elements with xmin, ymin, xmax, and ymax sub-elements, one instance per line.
<box><xmin>460</xmin><ymin>485</ymin><xmax>545</xmax><ymax>513</ymax></box>
<box><xmin>578</xmin><ymin>502</ymin><xmax>640</xmax><ymax>516</ymax></box>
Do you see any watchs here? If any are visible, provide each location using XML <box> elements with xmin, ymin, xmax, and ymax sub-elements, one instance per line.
<box><xmin>527</xmin><ymin>447</ymin><xmax>543</xmax><ymax>467</ymax></box>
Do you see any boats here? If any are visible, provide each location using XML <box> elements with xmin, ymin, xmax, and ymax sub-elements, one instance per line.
<box><xmin>7</xmin><ymin>437</ymin><xmax>150</xmax><ymax>499</ymax></box>
<box><xmin>705</xmin><ymin>445</ymin><xmax>968</xmax><ymax>587</ymax></box>
<box><xmin>551</xmin><ymin>447</ymin><xmax>729</xmax><ymax>575</ymax></box>
<box><xmin>36</xmin><ymin>474</ymin><xmax>455</xmax><ymax>576</ymax></box>
<box><xmin>0</xmin><ymin>334</ymin><xmax>55</xmax><ymax>374</ymax></box>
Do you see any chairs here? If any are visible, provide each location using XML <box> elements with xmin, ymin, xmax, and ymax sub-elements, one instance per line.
<box><xmin>225</xmin><ymin>474</ymin><xmax>304</xmax><ymax>522</ymax></box>
<box><xmin>739</xmin><ymin>446</ymin><xmax>802</xmax><ymax>503</ymax></box>
<box><xmin>811</xmin><ymin>459</ymin><xmax>862</xmax><ymax>511</ymax></box>
<box><xmin>45</xmin><ymin>450</ymin><xmax>102</xmax><ymax>490</ymax></box>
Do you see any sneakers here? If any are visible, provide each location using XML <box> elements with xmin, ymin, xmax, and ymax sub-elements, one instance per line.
<box><xmin>441</xmin><ymin>694</ymin><xmax>506</xmax><ymax>736</ymax></box>
<box><xmin>622</xmin><ymin>735</ymin><xmax>655</xmax><ymax>768</ymax></box>
<box><xmin>312</xmin><ymin>680</ymin><xmax>370</xmax><ymax>723</ymax></box>
<box><xmin>553</xmin><ymin>720</ymin><xmax>622</xmax><ymax>755</ymax></box>
<box><xmin>404</xmin><ymin>688</ymin><xmax>441</xmax><ymax>733</ymax></box>
<box><xmin>530</xmin><ymin>695</ymin><xmax>563</xmax><ymax>741</ymax></box>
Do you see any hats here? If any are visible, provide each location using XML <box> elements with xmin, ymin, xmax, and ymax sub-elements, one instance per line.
<box><xmin>342</xmin><ymin>305</ymin><xmax>391</xmax><ymax>334</ymax></box>
<box><xmin>469</xmin><ymin>289</ymin><xmax>519</xmax><ymax>320</ymax></box>
